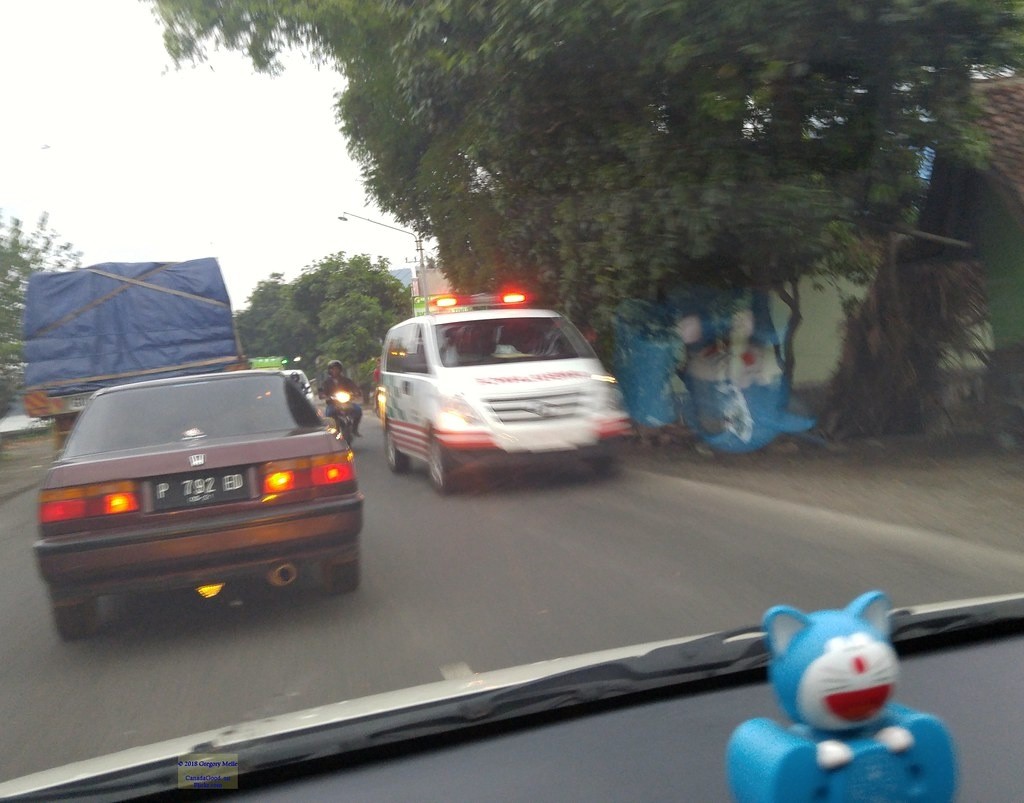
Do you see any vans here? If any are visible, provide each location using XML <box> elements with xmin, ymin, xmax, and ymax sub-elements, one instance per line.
<box><xmin>375</xmin><ymin>289</ymin><xmax>633</xmax><ymax>494</ymax></box>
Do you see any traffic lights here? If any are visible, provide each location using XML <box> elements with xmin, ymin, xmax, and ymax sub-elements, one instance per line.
<box><xmin>281</xmin><ymin>357</ymin><xmax>288</xmax><ymax>367</ymax></box>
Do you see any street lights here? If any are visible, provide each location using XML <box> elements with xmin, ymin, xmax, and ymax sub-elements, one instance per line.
<box><xmin>338</xmin><ymin>212</ymin><xmax>430</xmax><ymax>314</ymax></box>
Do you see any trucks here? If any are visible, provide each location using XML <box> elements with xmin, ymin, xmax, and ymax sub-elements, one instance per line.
<box><xmin>23</xmin><ymin>254</ymin><xmax>253</xmax><ymax>452</ymax></box>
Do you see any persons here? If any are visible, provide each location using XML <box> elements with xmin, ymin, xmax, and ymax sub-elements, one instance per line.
<box><xmin>290</xmin><ymin>372</ymin><xmax>308</xmax><ymax>394</ymax></box>
<box><xmin>455</xmin><ymin>327</ymin><xmax>489</xmax><ymax>361</ymax></box>
<box><xmin>374</xmin><ymin>360</ymin><xmax>380</xmax><ymax>413</ymax></box>
<box><xmin>317</xmin><ymin>360</ymin><xmax>363</xmax><ymax>438</ymax></box>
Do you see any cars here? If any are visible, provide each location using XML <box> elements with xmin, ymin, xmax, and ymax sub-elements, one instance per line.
<box><xmin>33</xmin><ymin>369</ymin><xmax>367</xmax><ymax>640</ymax></box>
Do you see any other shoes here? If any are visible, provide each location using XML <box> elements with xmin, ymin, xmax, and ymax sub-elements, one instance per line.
<box><xmin>352</xmin><ymin>429</ymin><xmax>361</xmax><ymax>437</ymax></box>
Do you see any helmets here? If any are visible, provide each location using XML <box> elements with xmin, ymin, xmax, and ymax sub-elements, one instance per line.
<box><xmin>327</xmin><ymin>360</ymin><xmax>342</xmax><ymax>374</ymax></box>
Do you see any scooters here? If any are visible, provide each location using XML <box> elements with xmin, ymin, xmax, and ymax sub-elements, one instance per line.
<box><xmin>318</xmin><ymin>388</ymin><xmax>360</xmax><ymax>442</ymax></box>
